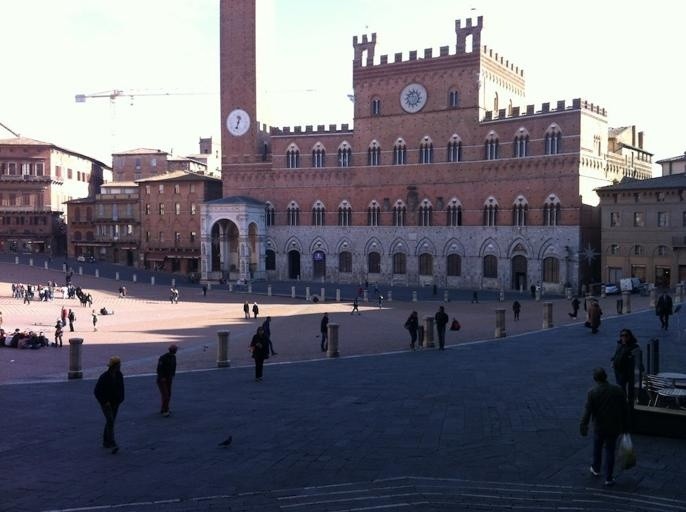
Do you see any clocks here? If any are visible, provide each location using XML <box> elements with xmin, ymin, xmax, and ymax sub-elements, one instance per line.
<box><xmin>224</xmin><ymin>108</ymin><xmax>252</xmax><ymax>138</ymax></box>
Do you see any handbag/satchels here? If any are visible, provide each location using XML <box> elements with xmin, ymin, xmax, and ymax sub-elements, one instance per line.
<box><xmin>251</xmin><ymin>347</ymin><xmax>255</xmax><ymax>358</ymax></box>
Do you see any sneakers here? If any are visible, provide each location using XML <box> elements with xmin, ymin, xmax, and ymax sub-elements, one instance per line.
<box><xmin>606</xmin><ymin>479</ymin><xmax>615</xmax><ymax>484</ymax></box>
<box><xmin>590</xmin><ymin>467</ymin><xmax>600</xmax><ymax>476</ymax></box>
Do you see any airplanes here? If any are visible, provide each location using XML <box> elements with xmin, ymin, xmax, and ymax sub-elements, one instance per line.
<box><xmin>75</xmin><ymin>89</ymin><xmax>170</xmax><ymax>107</ymax></box>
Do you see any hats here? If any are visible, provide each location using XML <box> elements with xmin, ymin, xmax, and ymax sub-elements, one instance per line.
<box><xmin>168</xmin><ymin>344</ymin><xmax>177</xmax><ymax>351</ymax></box>
<box><xmin>107</xmin><ymin>356</ymin><xmax>120</xmax><ymax>366</ymax></box>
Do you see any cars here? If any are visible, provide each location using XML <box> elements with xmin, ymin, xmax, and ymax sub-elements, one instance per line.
<box><xmin>604</xmin><ymin>276</ymin><xmax>657</xmax><ymax>297</ymax></box>
<box><xmin>74</xmin><ymin>254</ymin><xmax>96</xmax><ymax>263</ymax></box>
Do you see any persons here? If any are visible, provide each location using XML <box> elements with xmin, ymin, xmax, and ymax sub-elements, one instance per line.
<box><xmin>156</xmin><ymin>344</ymin><xmax>178</xmax><ymax>417</ymax></box>
<box><xmin>535</xmin><ymin>281</ymin><xmax>541</xmax><ymax>301</ymax></box>
<box><xmin>93</xmin><ymin>355</ymin><xmax>125</xmax><ymax>450</ymax></box>
<box><xmin>404</xmin><ymin>305</ymin><xmax>461</xmax><ymax>352</ymax></box>
<box><xmin>531</xmin><ymin>284</ymin><xmax>535</xmax><ymax>299</ymax></box>
<box><xmin>0</xmin><ymin>272</ymin><xmax>128</xmax><ymax>350</ymax></box>
<box><xmin>513</xmin><ymin>301</ymin><xmax>521</xmax><ymax>320</ymax></box>
<box><xmin>471</xmin><ymin>291</ymin><xmax>479</xmax><ymax>304</ymax></box>
<box><xmin>579</xmin><ymin>367</ymin><xmax>629</xmax><ymax>486</ymax></box>
<box><xmin>250</xmin><ymin>327</ymin><xmax>269</xmax><ymax>382</ymax></box>
<box><xmin>320</xmin><ymin>312</ymin><xmax>328</xmax><ymax>352</ymax></box>
<box><xmin>656</xmin><ymin>290</ymin><xmax>673</xmax><ymax>331</ymax></box>
<box><xmin>252</xmin><ymin>302</ymin><xmax>259</xmax><ymax>319</ymax></box>
<box><xmin>616</xmin><ymin>291</ymin><xmax>623</xmax><ymax>314</ymax></box>
<box><xmin>262</xmin><ymin>317</ymin><xmax>278</xmax><ymax>358</ymax></box>
<box><xmin>244</xmin><ymin>300</ymin><xmax>250</xmax><ymax>320</ymax></box>
<box><xmin>351</xmin><ymin>279</ymin><xmax>387</xmax><ymax>316</ymax></box>
<box><xmin>570</xmin><ymin>291</ymin><xmax>603</xmax><ymax>333</ymax></box>
<box><xmin>611</xmin><ymin>328</ymin><xmax>642</xmax><ymax>412</ymax></box>
<box><xmin>170</xmin><ymin>288</ymin><xmax>179</xmax><ymax>304</ymax></box>
<box><xmin>202</xmin><ymin>286</ymin><xmax>207</xmax><ymax>296</ymax></box>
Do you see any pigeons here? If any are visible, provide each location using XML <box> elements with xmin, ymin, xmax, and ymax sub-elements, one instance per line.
<box><xmin>218</xmin><ymin>436</ymin><xmax>233</xmax><ymax>448</ymax></box>
